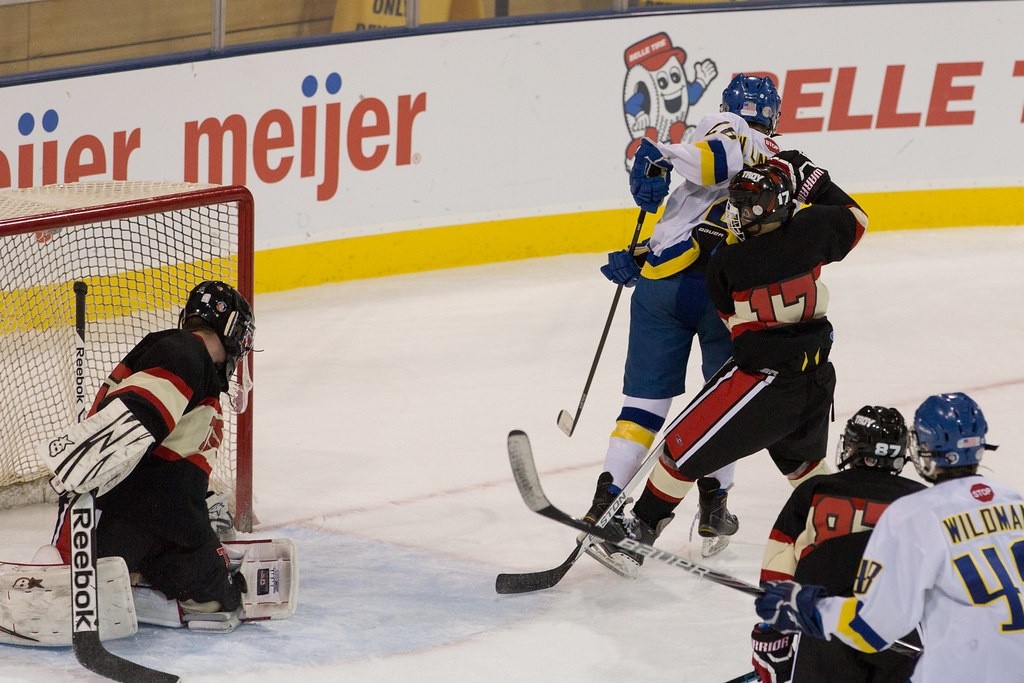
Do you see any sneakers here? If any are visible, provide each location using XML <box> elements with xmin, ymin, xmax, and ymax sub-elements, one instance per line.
<box><xmin>688</xmin><ymin>476</ymin><xmax>738</xmax><ymax>557</ymax></box>
<box><xmin>576</xmin><ymin>509</ymin><xmax>675</xmax><ymax>580</ymax></box>
<box><xmin>585</xmin><ymin>472</ymin><xmax>637</xmax><ymax>540</ymax></box>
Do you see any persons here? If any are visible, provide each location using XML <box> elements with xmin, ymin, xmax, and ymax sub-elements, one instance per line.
<box><xmin>574</xmin><ymin>74</ymin><xmax>786</xmax><ymax>550</ymax></box>
<box><xmin>580</xmin><ymin>151</ymin><xmax>872</xmax><ymax>580</ymax></box>
<box><xmin>756</xmin><ymin>391</ymin><xmax>1024</xmax><ymax>683</ymax></box>
<box><xmin>39</xmin><ymin>281</ymin><xmax>254</xmax><ymax>632</ymax></box>
<box><xmin>750</xmin><ymin>403</ymin><xmax>925</xmax><ymax>683</ymax></box>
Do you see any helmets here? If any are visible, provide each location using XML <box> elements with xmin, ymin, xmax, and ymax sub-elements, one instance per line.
<box><xmin>178</xmin><ymin>280</ymin><xmax>264</xmax><ymax>412</ymax></box>
<box><xmin>834</xmin><ymin>405</ymin><xmax>909</xmax><ymax>476</ymax></box>
<box><xmin>906</xmin><ymin>392</ymin><xmax>998</xmax><ymax>484</ymax></box>
<box><xmin>720</xmin><ymin>72</ymin><xmax>782</xmax><ymax>138</ymax></box>
<box><xmin>725</xmin><ymin>164</ymin><xmax>795</xmax><ymax>242</ymax></box>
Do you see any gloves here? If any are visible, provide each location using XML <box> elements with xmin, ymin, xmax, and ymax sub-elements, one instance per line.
<box><xmin>766</xmin><ymin>150</ymin><xmax>828</xmax><ymax>204</ymax></box>
<box><xmin>600</xmin><ymin>249</ymin><xmax>643</xmax><ymax>288</ymax></box>
<box><xmin>629</xmin><ymin>137</ymin><xmax>674</xmax><ymax>214</ymax></box>
<box><xmin>754</xmin><ymin>579</ymin><xmax>832</xmax><ymax>643</ymax></box>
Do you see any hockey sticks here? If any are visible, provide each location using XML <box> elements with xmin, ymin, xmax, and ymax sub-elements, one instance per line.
<box><xmin>555</xmin><ymin>164</ymin><xmax>662</xmax><ymax>440</ymax></box>
<box><xmin>66</xmin><ymin>276</ymin><xmax>184</xmax><ymax>683</ymax></box>
<box><xmin>506</xmin><ymin>428</ymin><xmax>922</xmax><ymax>660</ymax></box>
<box><xmin>493</xmin><ymin>354</ymin><xmax>737</xmax><ymax>597</ymax></box>
